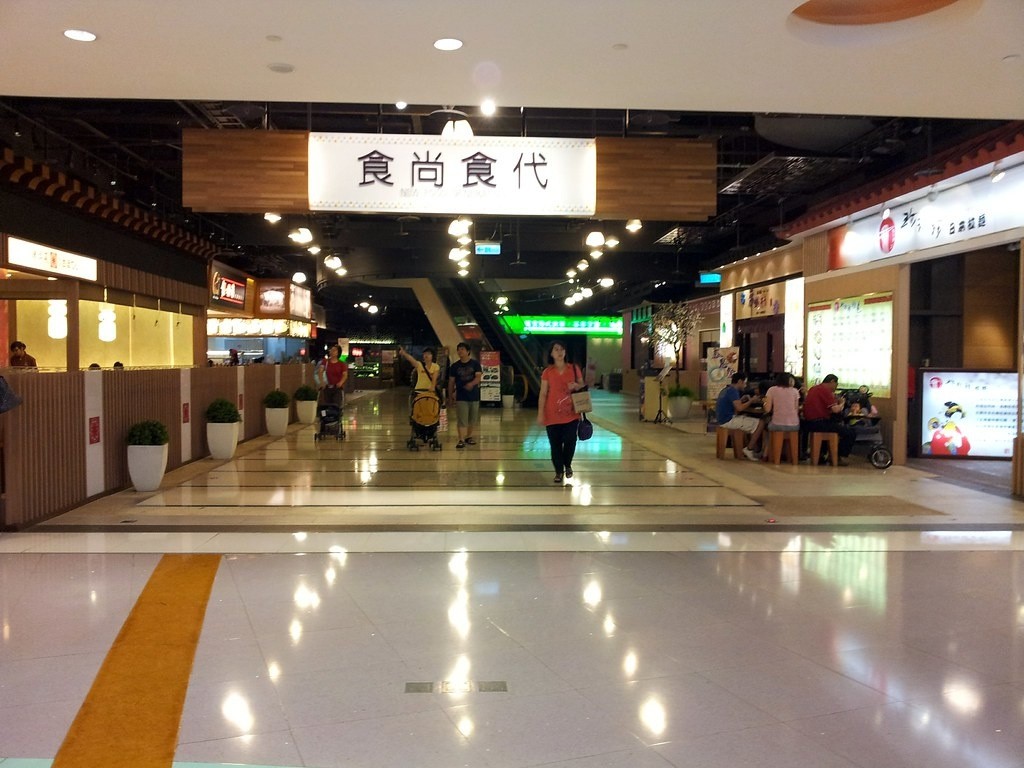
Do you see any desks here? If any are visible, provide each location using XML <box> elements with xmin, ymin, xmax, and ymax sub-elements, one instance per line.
<box><xmin>737</xmin><ymin>402</ymin><xmax>803</xmax><ymax>419</ymax></box>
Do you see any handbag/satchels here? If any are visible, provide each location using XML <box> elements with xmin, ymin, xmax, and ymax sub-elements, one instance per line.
<box><xmin>578</xmin><ymin>419</ymin><xmax>592</xmax><ymax>440</ymax></box>
<box><xmin>571</xmin><ymin>391</ymin><xmax>593</xmax><ymax>414</ymax></box>
<box><xmin>436</xmin><ymin>387</ymin><xmax>444</xmax><ymax>405</ymax></box>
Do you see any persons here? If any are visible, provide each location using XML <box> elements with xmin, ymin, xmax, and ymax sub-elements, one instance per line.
<box><xmin>448</xmin><ymin>342</ymin><xmax>481</xmax><ymax>448</ymax></box>
<box><xmin>716</xmin><ymin>371</ymin><xmax>857</xmax><ymax>465</ymax></box>
<box><xmin>228</xmin><ymin>349</ymin><xmax>239</xmax><ymax>366</ymax></box>
<box><xmin>88</xmin><ymin>364</ymin><xmax>101</xmax><ymax>370</ymax></box>
<box><xmin>114</xmin><ymin>362</ymin><xmax>123</xmax><ymax>370</ymax></box>
<box><xmin>537</xmin><ymin>340</ymin><xmax>585</xmax><ymax>483</ymax></box>
<box><xmin>319</xmin><ymin>344</ymin><xmax>348</xmax><ymax>390</ymax></box>
<box><xmin>11</xmin><ymin>342</ymin><xmax>38</xmax><ymax>370</ymax></box>
<box><xmin>399</xmin><ymin>347</ymin><xmax>440</xmax><ymax>394</ymax></box>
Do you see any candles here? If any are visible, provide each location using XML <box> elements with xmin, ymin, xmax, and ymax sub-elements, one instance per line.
<box><xmin>126</xmin><ymin>419</ymin><xmax>170</xmax><ymax>491</ymax></box>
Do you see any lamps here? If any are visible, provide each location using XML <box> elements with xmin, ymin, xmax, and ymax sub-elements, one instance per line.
<box><xmin>263</xmin><ymin>105</ymin><xmax>644</xmax><ymax>314</ymax></box>
<box><xmin>700</xmin><ymin>270</ymin><xmax>722</xmax><ymax>284</ymax></box>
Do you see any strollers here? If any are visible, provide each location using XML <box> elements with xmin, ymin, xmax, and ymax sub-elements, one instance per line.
<box><xmin>313</xmin><ymin>384</ymin><xmax>346</xmax><ymax>441</ymax></box>
<box><xmin>406</xmin><ymin>389</ymin><xmax>442</xmax><ymax>451</ymax></box>
<box><xmin>840</xmin><ymin>389</ymin><xmax>893</xmax><ymax>468</ymax></box>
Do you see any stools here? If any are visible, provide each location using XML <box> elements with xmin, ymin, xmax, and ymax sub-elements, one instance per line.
<box><xmin>716</xmin><ymin>425</ymin><xmax>840</xmax><ymax>467</ymax></box>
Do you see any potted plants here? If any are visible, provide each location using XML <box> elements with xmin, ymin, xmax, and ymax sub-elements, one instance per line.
<box><xmin>638</xmin><ymin>300</ymin><xmax>706</xmax><ymax>419</ymax></box>
<box><xmin>204</xmin><ymin>399</ymin><xmax>243</xmax><ymax>460</ymax></box>
<box><xmin>294</xmin><ymin>385</ymin><xmax>318</xmax><ymax>423</ymax></box>
<box><xmin>500</xmin><ymin>383</ymin><xmax>515</xmax><ymax>409</ymax></box>
<box><xmin>262</xmin><ymin>389</ymin><xmax>292</xmax><ymax>437</ymax></box>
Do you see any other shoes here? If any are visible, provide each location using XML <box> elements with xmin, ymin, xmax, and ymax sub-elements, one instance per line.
<box><xmin>565</xmin><ymin>464</ymin><xmax>572</xmax><ymax>478</ymax></box>
<box><xmin>743</xmin><ymin>447</ymin><xmax>758</xmax><ymax>461</ymax></box>
<box><xmin>554</xmin><ymin>472</ymin><xmax>565</xmax><ymax>482</ymax></box>
<box><xmin>838</xmin><ymin>457</ymin><xmax>849</xmax><ymax>466</ymax></box>
<box><xmin>760</xmin><ymin>452</ymin><xmax>767</xmax><ymax>461</ymax></box>
<box><xmin>466</xmin><ymin>438</ymin><xmax>476</xmax><ymax>446</ymax></box>
<box><xmin>456</xmin><ymin>441</ymin><xmax>464</xmax><ymax>448</ymax></box>
